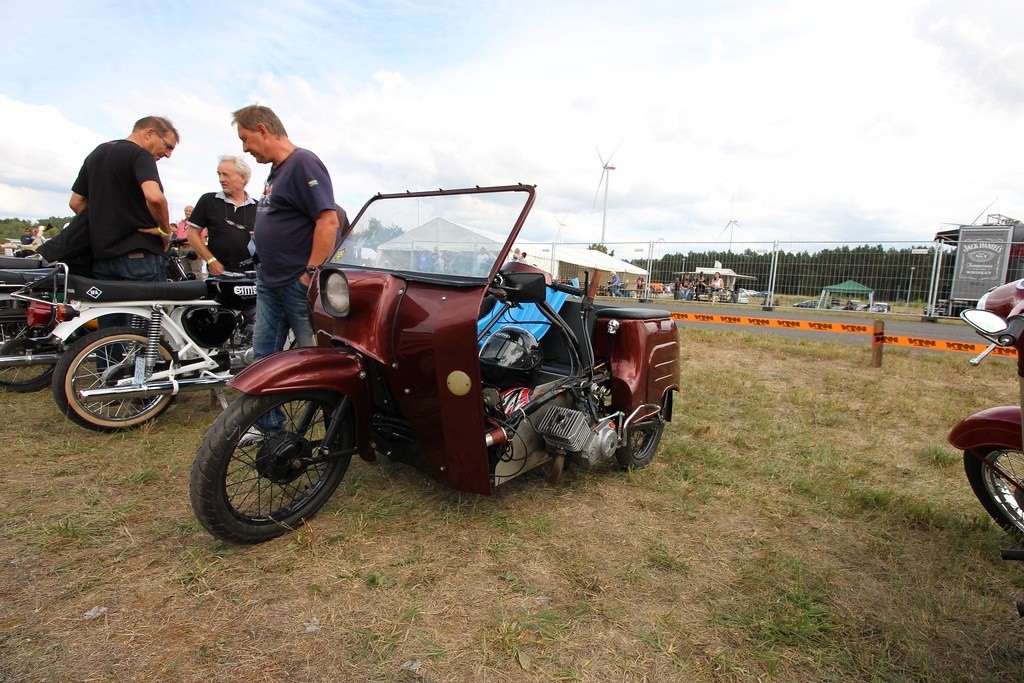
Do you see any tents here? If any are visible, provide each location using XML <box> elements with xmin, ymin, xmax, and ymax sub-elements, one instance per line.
<box><xmin>818</xmin><ymin>280</ymin><xmax>875</xmax><ymax>312</ymax></box>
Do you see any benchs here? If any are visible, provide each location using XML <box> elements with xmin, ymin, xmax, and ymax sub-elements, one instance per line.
<box><xmin>705</xmin><ymin>286</ymin><xmax>730</xmax><ymax>303</ymax></box>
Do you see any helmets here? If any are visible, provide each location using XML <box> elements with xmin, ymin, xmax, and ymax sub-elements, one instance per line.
<box><xmin>478</xmin><ymin>326</ymin><xmax>541</xmax><ymax>386</ymax></box>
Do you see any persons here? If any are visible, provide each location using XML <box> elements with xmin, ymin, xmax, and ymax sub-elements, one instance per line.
<box><xmin>170</xmin><ymin>205</ymin><xmax>208</xmax><ymax>280</ymax></box>
<box><xmin>414</xmin><ymin>246</ymin><xmax>527</xmax><ymax>277</ymax></box>
<box><xmin>187</xmin><ymin>154</ymin><xmax>259</xmax><ymax>318</ymax></box>
<box><xmin>674</xmin><ymin>271</ymin><xmax>742</xmax><ymax>305</ymax></box>
<box><xmin>610</xmin><ymin>271</ymin><xmax>623</xmax><ymax>297</ymax></box>
<box><xmin>636</xmin><ymin>275</ymin><xmax>645</xmax><ymax>295</ymax></box>
<box><xmin>20</xmin><ymin>225</ymin><xmax>46</xmax><ymax>258</ymax></box>
<box><xmin>831</xmin><ymin>296</ymin><xmax>891</xmax><ymax>313</ymax></box>
<box><xmin>231</xmin><ymin>103</ymin><xmax>361</xmax><ymax>449</ymax></box>
<box><xmin>69</xmin><ymin>115</ymin><xmax>180</xmax><ymax>406</ymax></box>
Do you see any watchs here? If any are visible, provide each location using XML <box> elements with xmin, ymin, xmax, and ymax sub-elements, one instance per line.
<box><xmin>305</xmin><ymin>265</ymin><xmax>316</xmax><ymax>277</ymax></box>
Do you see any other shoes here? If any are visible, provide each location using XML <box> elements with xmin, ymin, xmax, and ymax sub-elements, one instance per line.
<box><xmin>239</xmin><ymin>428</ymin><xmax>271</xmax><ymax>447</ymax></box>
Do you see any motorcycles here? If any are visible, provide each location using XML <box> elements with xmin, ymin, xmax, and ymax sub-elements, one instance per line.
<box><xmin>191</xmin><ymin>183</ymin><xmax>682</xmax><ymax>545</ymax></box>
<box><xmin>0</xmin><ymin>236</ymin><xmax>196</xmax><ymax>392</ymax></box>
<box><xmin>948</xmin><ymin>274</ymin><xmax>1024</xmax><ymax>563</ymax></box>
<box><xmin>12</xmin><ymin>264</ymin><xmax>256</xmax><ymax>432</ymax></box>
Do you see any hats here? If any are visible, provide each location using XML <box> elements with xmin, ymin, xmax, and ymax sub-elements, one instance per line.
<box><xmin>611</xmin><ymin>271</ymin><xmax>616</xmax><ymax>275</ymax></box>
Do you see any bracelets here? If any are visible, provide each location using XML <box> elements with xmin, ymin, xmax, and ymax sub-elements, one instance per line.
<box><xmin>157</xmin><ymin>225</ymin><xmax>172</xmax><ymax>237</ymax></box>
<box><xmin>207</xmin><ymin>257</ymin><xmax>217</xmax><ymax>265</ymax></box>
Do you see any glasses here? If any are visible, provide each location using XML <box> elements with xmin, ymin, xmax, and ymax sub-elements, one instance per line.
<box><xmin>153</xmin><ymin>130</ymin><xmax>172</xmax><ymax>151</ymax></box>
<box><xmin>224</xmin><ymin>217</ymin><xmax>247</xmax><ymax>230</ymax></box>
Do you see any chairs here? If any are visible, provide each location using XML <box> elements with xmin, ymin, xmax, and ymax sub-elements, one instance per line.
<box><xmin>477</xmin><ymin>286</ymin><xmax>572</xmax><ymax>354</ymax></box>
